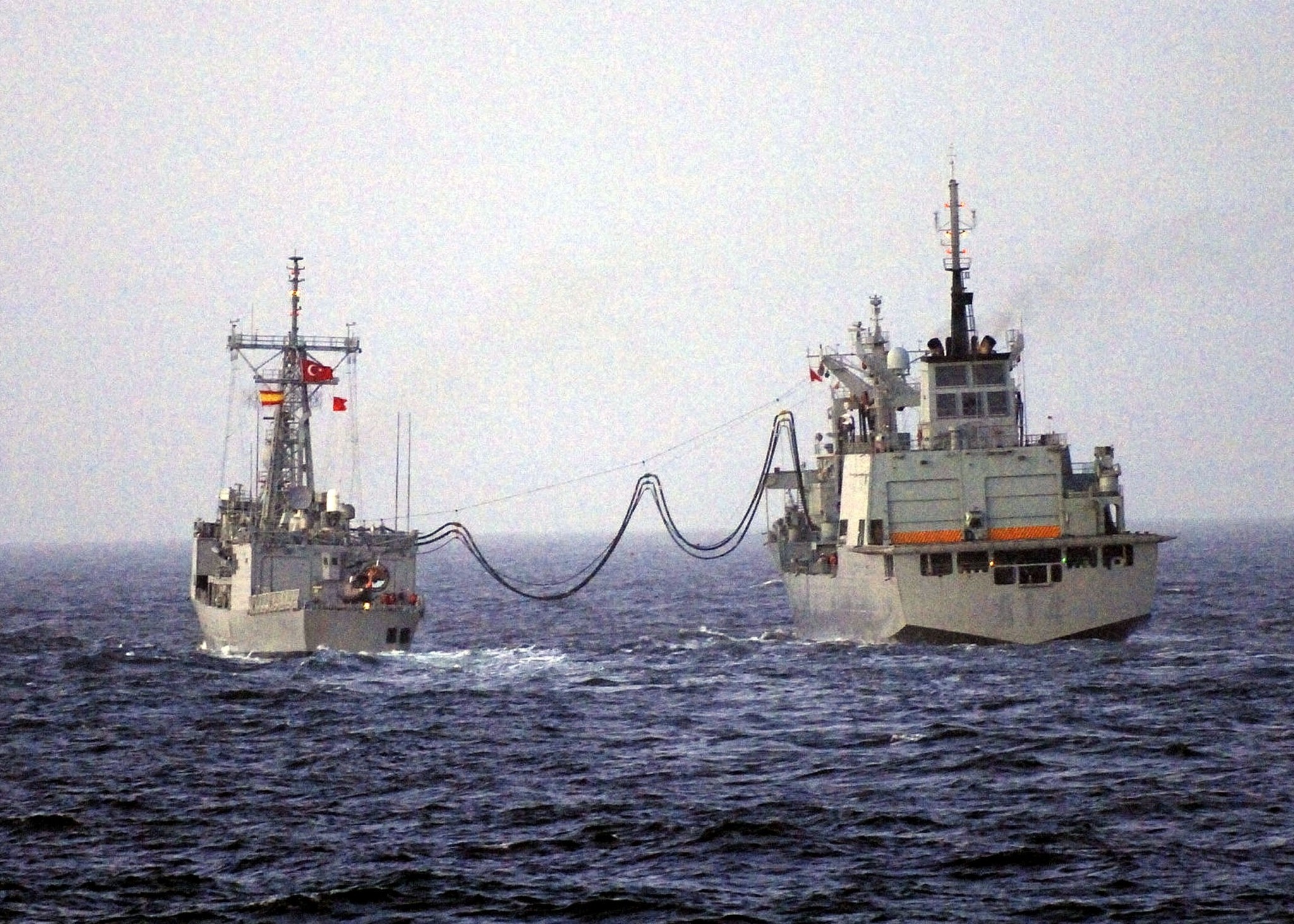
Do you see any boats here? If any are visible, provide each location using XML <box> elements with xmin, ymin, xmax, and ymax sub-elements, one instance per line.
<box><xmin>178</xmin><ymin>248</ymin><xmax>424</xmax><ymax>654</ymax></box>
<box><xmin>758</xmin><ymin>157</ymin><xmax>1176</xmax><ymax>650</ymax></box>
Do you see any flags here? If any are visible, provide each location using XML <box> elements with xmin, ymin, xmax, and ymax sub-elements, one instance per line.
<box><xmin>260</xmin><ymin>391</ymin><xmax>285</xmax><ymax>406</ymax></box>
<box><xmin>333</xmin><ymin>397</ymin><xmax>348</xmax><ymax>411</ymax></box>
<box><xmin>300</xmin><ymin>358</ymin><xmax>333</xmax><ymax>382</ymax></box>
<box><xmin>809</xmin><ymin>368</ymin><xmax>822</xmax><ymax>382</ymax></box>
